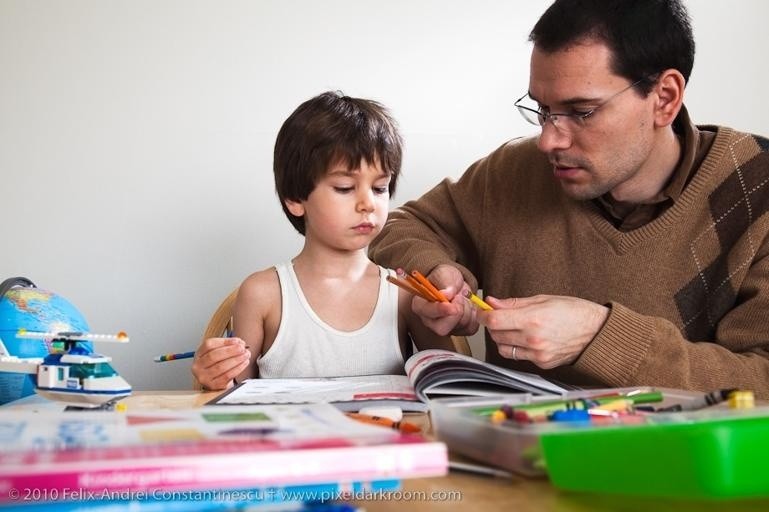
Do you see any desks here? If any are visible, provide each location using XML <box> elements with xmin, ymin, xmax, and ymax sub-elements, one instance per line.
<box><xmin>0</xmin><ymin>388</ymin><xmax>769</xmax><ymax>512</ymax></box>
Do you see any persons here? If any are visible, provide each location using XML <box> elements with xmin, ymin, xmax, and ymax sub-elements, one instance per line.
<box><xmin>365</xmin><ymin>2</ymin><xmax>769</xmax><ymax>406</ymax></box>
<box><xmin>190</xmin><ymin>89</ymin><xmax>473</xmax><ymax>393</ymax></box>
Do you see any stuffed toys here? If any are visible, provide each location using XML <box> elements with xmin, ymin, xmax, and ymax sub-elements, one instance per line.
<box><xmin>0</xmin><ymin>317</ymin><xmax>139</xmax><ymax>410</ymax></box>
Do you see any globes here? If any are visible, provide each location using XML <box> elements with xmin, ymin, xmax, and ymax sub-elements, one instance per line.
<box><xmin>0</xmin><ymin>276</ymin><xmax>93</xmax><ymax>404</ymax></box>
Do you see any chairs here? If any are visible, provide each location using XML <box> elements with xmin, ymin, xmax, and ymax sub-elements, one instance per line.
<box><xmin>203</xmin><ymin>286</ymin><xmax>237</xmax><ymax>338</ymax></box>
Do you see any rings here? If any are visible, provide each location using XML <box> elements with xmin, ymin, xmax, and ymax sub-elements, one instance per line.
<box><xmin>511</xmin><ymin>344</ymin><xmax>519</xmax><ymax>362</ymax></box>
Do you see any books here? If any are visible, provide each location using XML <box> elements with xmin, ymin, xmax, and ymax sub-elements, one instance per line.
<box><xmin>206</xmin><ymin>343</ymin><xmax>570</xmax><ymax>418</ymax></box>
<box><xmin>0</xmin><ymin>392</ymin><xmax>451</xmax><ymax>511</ymax></box>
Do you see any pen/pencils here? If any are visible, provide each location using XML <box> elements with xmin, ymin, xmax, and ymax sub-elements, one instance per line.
<box><xmin>346</xmin><ymin>412</ymin><xmax>422</xmax><ymax>434</ymax></box>
<box><xmin>154</xmin><ymin>345</ymin><xmax>250</xmax><ymax>362</ymax></box>
<box><xmin>468</xmin><ymin>389</ymin><xmax>740</xmax><ymax>426</ymax></box>
<box><xmin>386</xmin><ymin>267</ymin><xmax>497</xmax><ymax>311</ymax></box>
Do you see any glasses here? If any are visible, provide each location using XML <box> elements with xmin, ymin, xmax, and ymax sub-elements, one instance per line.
<box><xmin>513</xmin><ymin>70</ymin><xmax>662</xmax><ymax>134</ymax></box>
